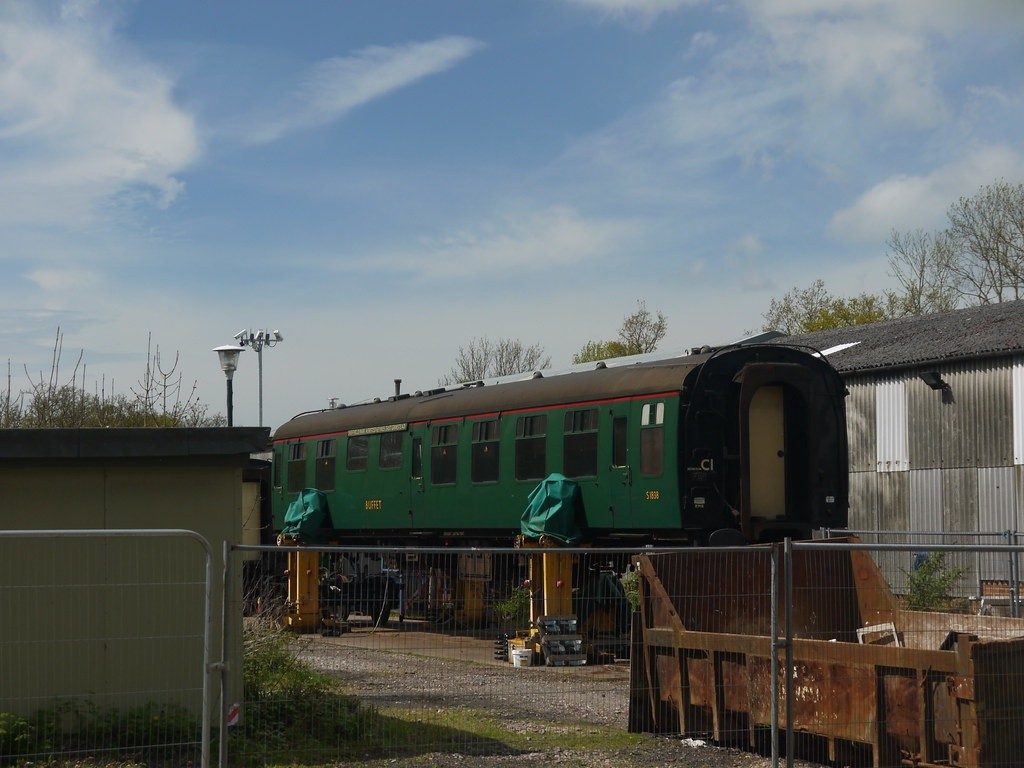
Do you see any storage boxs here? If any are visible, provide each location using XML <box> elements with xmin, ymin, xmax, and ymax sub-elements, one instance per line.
<box><xmin>537</xmin><ymin>614</ymin><xmax>587</xmax><ymax>666</ymax></box>
<box><xmin>508</xmin><ymin>637</ymin><xmax>536</xmax><ymax>663</ymax></box>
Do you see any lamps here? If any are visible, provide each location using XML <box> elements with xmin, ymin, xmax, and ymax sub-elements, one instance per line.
<box><xmin>919</xmin><ymin>371</ymin><xmax>944</xmax><ymax>390</ymax></box>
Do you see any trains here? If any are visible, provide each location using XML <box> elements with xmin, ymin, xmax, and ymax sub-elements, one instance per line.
<box><xmin>271</xmin><ymin>340</ymin><xmax>852</xmax><ymax>630</ymax></box>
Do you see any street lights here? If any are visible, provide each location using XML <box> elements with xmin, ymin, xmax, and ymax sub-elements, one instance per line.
<box><xmin>235</xmin><ymin>329</ymin><xmax>282</xmax><ymax>427</ymax></box>
<box><xmin>213</xmin><ymin>348</ymin><xmax>245</xmax><ymax>427</ymax></box>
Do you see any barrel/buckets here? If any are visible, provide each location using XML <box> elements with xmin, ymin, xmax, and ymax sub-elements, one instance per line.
<box><xmin>512</xmin><ymin>649</ymin><xmax>532</xmax><ymax>667</ymax></box>
<box><xmin>508</xmin><ymin>640</ymin><xmax>525</xmax><ymax>664</ymax></box>
